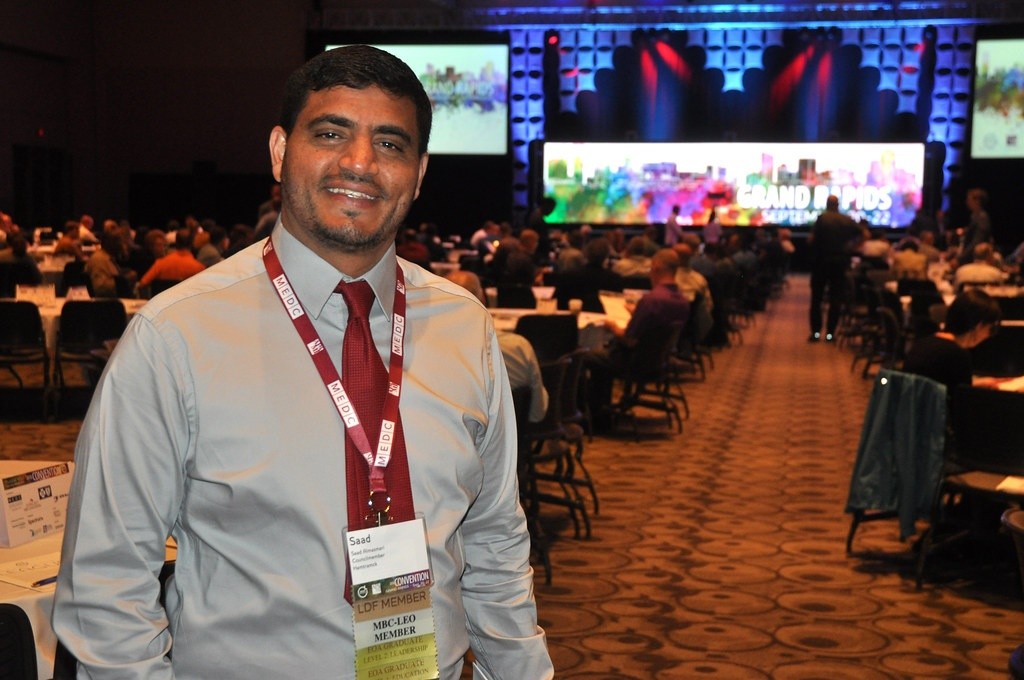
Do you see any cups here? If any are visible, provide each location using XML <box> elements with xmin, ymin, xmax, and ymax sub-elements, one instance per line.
<box><xmin>570</xmin><ymin>299</ymin><xmax>582</xmax><ymax>315</ymax></box>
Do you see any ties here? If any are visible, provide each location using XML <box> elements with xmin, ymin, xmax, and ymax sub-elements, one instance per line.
<box><xmin>333</xmin><ymin>279</ymin><xmax>416</xmax><ymax>607</ymax></box>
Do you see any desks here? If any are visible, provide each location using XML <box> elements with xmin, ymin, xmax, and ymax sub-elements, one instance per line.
<box><xmin>0</xmin><ymin>257</ymin><xmax>148</xmax><ymax>388</ymax></box>
<box><xmin>431</xmin><ymin>256</ymin><xmax>649</xmax><ymax>335</ymax></box>
<box><xmin>0</xmin><ymin>460</ymin><xmax>177</xmax><ymax>680</ymax></box>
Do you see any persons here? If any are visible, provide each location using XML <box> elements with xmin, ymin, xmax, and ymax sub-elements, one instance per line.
<box><xmin>841</xmin><ymin>188</ymin><xmax>1024</xmax><ymax>579</ymax></box>
<box><xmin>0</xmin><ymin>181</ymin><xmax>796</xmax><ymax>435</ymax></box>
<box><xmin>49</xmin><ymin>41</ymin><xmax>554</xmax><ymax>680</ymax></box>
<box><xmin>808</xmin><ymin>195</ymin><xmax>861</xmax><ymax>342</ymax></box>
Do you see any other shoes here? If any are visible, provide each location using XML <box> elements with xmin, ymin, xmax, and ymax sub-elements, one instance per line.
<box><xmin>826</xmin><ymin>336</ymin><xmax>836</xmax><ymax>342</ymax></box>
<box><xmin>808</xmin><ymin>334</ymin><xmax>819</xmax><ymax>342</ymax></box>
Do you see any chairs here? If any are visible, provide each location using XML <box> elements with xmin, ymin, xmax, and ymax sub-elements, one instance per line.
<box><xmin>51</xmin><ymin>300</ymin><xmax>128</xmax><ymax>420</ymax></box>
<box><xmin>0</xmin><ymin>298</ymin><xmax>52</xmax><ymax>417</ymax></box>
<box><xmin>829</xmin><ymin>260</ymin><xmax>1024</xmax><ymax>595</ymax></box>
<box><xmin>513</xmin><ymin>250</ymin><xmax>793</xmax><ymax>587</ymax></box>
